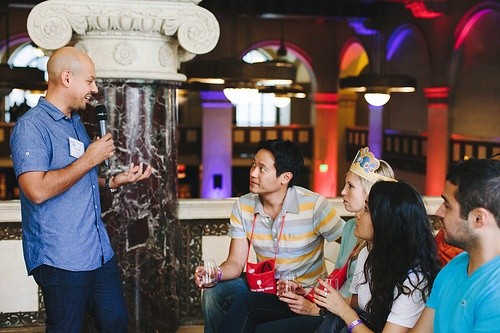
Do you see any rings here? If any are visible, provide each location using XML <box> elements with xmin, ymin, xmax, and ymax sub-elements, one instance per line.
<box><xmin>324</xmin><ymin>293</ymin><xmax>328</xmax><ymax>297</ymax></box>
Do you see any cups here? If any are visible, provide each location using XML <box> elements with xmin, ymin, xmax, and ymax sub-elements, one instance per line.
<box><xmin>279</xmin><ymin>271</ymin><xmax>299</xmax><ymax>297</ymax></box>
<box><xmin>199</xmin><ymin>259</ymin><xmax>219</xmax><ymax>288</ymax></box>
<box><xmin>313</xmin><ymin>273</ymin><xmax>338</xmax><ymax>303</ymax></box>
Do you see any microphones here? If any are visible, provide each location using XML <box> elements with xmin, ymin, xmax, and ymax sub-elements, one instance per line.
<box><xmin>95</xmin><ymin>104</ymin><xmax>109</xmax><ymax>166</ymax></box>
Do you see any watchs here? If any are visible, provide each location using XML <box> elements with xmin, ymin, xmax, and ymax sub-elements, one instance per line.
<box><xmin>105</xmin><ymin>179</ymin><xmax>117</xmax><ymax>193</ymax></box>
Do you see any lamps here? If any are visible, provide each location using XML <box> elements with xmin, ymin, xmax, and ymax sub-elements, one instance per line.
<box><xmin>339</xmin><ymin>75</ymin><xmax>417</xmax><ymax>107</ymax></box>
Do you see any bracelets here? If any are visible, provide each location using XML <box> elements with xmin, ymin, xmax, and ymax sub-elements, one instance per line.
<box><xmin>348</xmin><ymin>319</ymin><xmax>363</xmax><ymax>333</ymax></box>
<box><xmin>218</xmin><ymin>267</ymin><xmax>222</xmax><ymax>280</ymax></box>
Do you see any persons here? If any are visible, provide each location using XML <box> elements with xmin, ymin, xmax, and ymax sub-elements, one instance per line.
<box><xmin>9</xmin><ymin>45</ymin><xmax>152</xmax><ymax>333</ymax></box>
<box><xmin>313</xmin><ymin>181</ymin><xmax>442</xmax><ymax>333</ymax></box>
<box><xmin>193</xmin><ymin>139</ymin><xmax>347</xmax><ymax>333</ymax></box>
<box><xmin>223</xmin><ymin>147</ymin><xmax>395</xmax><ymax>333</ymax></box>
<box><xmin>408</xmin><ymin>159</ymin><xmax>500</xmax><ymax>333</ymax></box>
